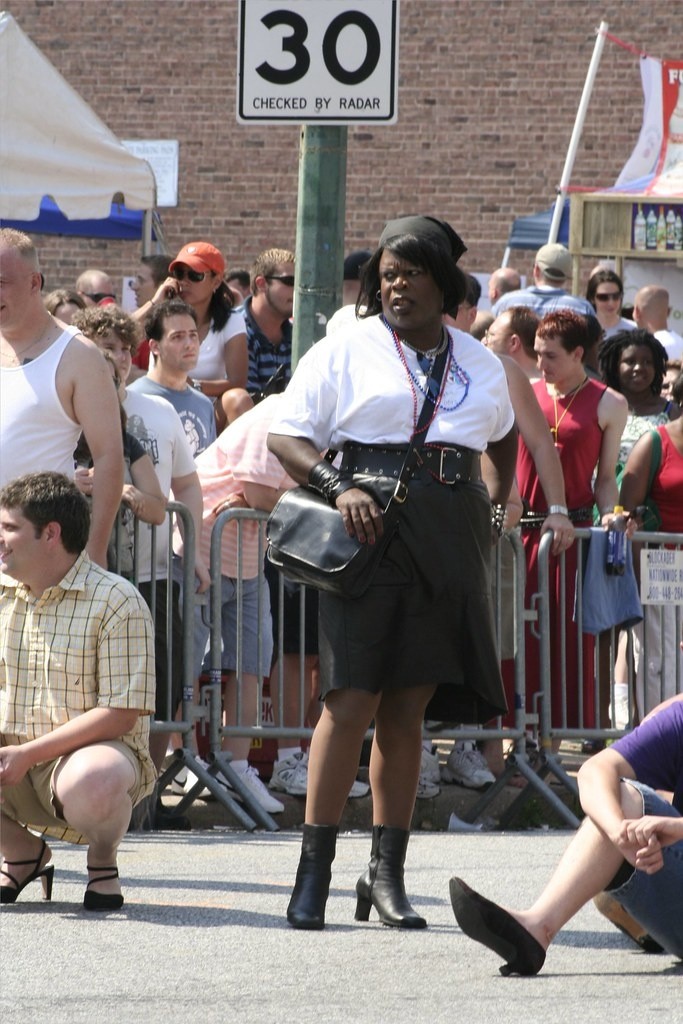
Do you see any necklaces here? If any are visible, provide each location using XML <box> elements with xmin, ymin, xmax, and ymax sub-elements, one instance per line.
<box><xmin>550</xmin><ymin>374</ymin><xmax>588</xmax><ymax>447</ymax></box>
<box><xmin>380</xmin><ymin>314</ymin><xmax>470</xmax><ymax>433</ymax></box>
<box><xmin>0</xmin><ymin>310</ymin><xmax>51</xmax><ymax>362</ymax></box>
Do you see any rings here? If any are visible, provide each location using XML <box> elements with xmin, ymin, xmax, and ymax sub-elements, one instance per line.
<box><xmin>568</xmin><ymin>536</ymin><xmax>573</xmax><ymax>541</ymax></box>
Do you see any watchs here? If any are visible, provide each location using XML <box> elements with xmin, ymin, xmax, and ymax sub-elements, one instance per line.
<box><xmin>192</xmin><ymin>377</ymin><xmax>201</xmax><ymax>392</ymax></box>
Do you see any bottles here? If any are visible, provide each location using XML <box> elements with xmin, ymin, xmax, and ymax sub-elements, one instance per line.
<box><xmin>634</xmin><ymin>203</ymin><xmax>683</xmax><ymax>251</ymax></box>
<box><xmin>605</xmin><ymin>505</ymin><xmax>627</xmax><ymax>577</ymax></box>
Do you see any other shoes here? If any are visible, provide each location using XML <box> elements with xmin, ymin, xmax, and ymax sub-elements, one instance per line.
<box><xmin>582</xmin><ymin>738</ymin><xmax>606</xmax><ymax>753</ymax></box>
<box><xmin>416</xmin><ymin>777</ymin><xmax>441</xmax><ymax>799</ymax></box>
<box><xmin>170</xmin><ymin>763</ymin><xmax>212</xmax><ymax>797</ymax></box>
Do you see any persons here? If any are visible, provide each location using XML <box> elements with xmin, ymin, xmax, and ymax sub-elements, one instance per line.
<box><xmin>0</xmin><ymin>229</ymin><xmax>124</xmax><ymax>573</ymax></box>
<box><xmin>1</xmin><ymin>473</ymin><xmax>160</xmax><ymax>913</ymax></box>
<box><xmin>42</xmin><ymin>243</ymin><xmax>373</xmax><ymax>811</ymax></box>
<box><xmin>417</xmin><ymin>243</ymin><xmax>683</xmax><ymax>801</ymax></box>
<box><xmin>449</xmin><ymin>695</ymin><xmax>683</xmax><ymax>978</ymax></box>
<box><xmin>264</xmin><ymin>214</ymin><xmax>517</xmax><ymax>930</ymax></box>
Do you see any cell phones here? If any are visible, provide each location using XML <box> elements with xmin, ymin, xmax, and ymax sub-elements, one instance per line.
<box><xmin>166</xmin><ymin>289</ymin><xmax>175</xmax><ymax>299</ymax></box>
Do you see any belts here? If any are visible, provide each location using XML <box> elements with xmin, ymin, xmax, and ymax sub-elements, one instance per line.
<box><xmin>522</xmin><ymin>508</ymin><xmax>591</xmax><ymax>528</ymax></box>
<box><xmin>339</xmin><ymin>442</ymin><xmax>483</xmax><ymax>485</ymax></box>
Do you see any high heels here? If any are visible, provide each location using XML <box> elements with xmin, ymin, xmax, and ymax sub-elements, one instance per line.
<box><xmin>83</xmin><ymin>866</ymin><xmax>124</xmax><ymax>911</ymax></box>
<box><xmin>594</xmin><ymin>891</ymin><xmax>664</xmax><ymax>954</ymax></box>
<box><xmin>0</xmin><ymin>839</ymin><xmax>54</xmax><ymax>903</ymax></box>
<box><xmin>449</xmin><ymin>877</ymin><xmax>546</xmax><ymax>977</ymax></box>
<box><xmin>286</xmin><ymin>823</ymin><xmax>339</xmax><ymax>928</ymax></box>
<box><xmin>354</xmin><ymin>825</ymin><xmax>427</xmax><ymax>928</ymax></box>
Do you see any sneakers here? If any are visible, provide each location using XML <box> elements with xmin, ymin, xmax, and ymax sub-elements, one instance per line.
<box><xmin>213</xmin><ymin>766</ymin><xmax>284</xmax><ymax>812</ymax></box>
<box><xmin>269</xmin><ymin>752</ymin><xmax>304</xmax><ymax>790</ymax></box>
<box><xmin>420</xmin><ymin>745</ymin><xmax>441</xmax><ymax>781</ymax></box>
<box><xmin>286</xmin><ymin>754</ymin><xmax>370</xmax><ymax>797</ymax></box>
<box><xmin>441</xmin><ymin>748</ymin><xmax>496</xmax><ymax>789</ymax></box>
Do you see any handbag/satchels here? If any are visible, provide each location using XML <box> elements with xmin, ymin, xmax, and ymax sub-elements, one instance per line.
<box><xmin>265</xmin><ymin>486</ymin><xmax>396</xmax><ymax>600</ymax></box>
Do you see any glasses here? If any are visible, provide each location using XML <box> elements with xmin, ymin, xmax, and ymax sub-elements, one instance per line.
<box><xmin>173</xmin><ymin>270</ymin><xmax>204</xmax><ymax>282</ymax></box>
<box><xmin>82</xmin><ymin>292</ymin><xmax>115</xmax><ymax>303</ymax></box>
<box><xmin>597</xmin><ymin>293</ymin><xmax>619</xmax><ymax>301</ymax></box>
<box><xmin>269</xmin><ymin>276</ymin><xmax>294</xmax><ymax>286</ymax></box>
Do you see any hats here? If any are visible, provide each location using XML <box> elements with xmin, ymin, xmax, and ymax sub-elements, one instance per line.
<box><xmin>343</xmin><ymin>252</ymin><xmax>373</xmax><ymax>280</ymax></box>
<box><xmin>169</xmin><ymin>242</ymin><xmax>224</xmax><ymax>273</ymax></box>
<box><xmin>378</xmin><ymin>215</ymin><xmax>468</xmax><ymax>265</ymax></box>
<box><xmin>535</xmin><ymin>244</ymin><xmax>572</xmax><ymax>280</ymax></box>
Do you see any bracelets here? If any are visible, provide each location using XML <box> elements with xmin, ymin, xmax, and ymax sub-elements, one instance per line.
<box><xmin>549</xmin><ymin>505</ymin><xmax>568</xmax><ymax>516</ymax></box>
<box><xmin>149</xmin><ymin>298</ymin><xmax>155</xmax><ymax>306</ymax></box>
